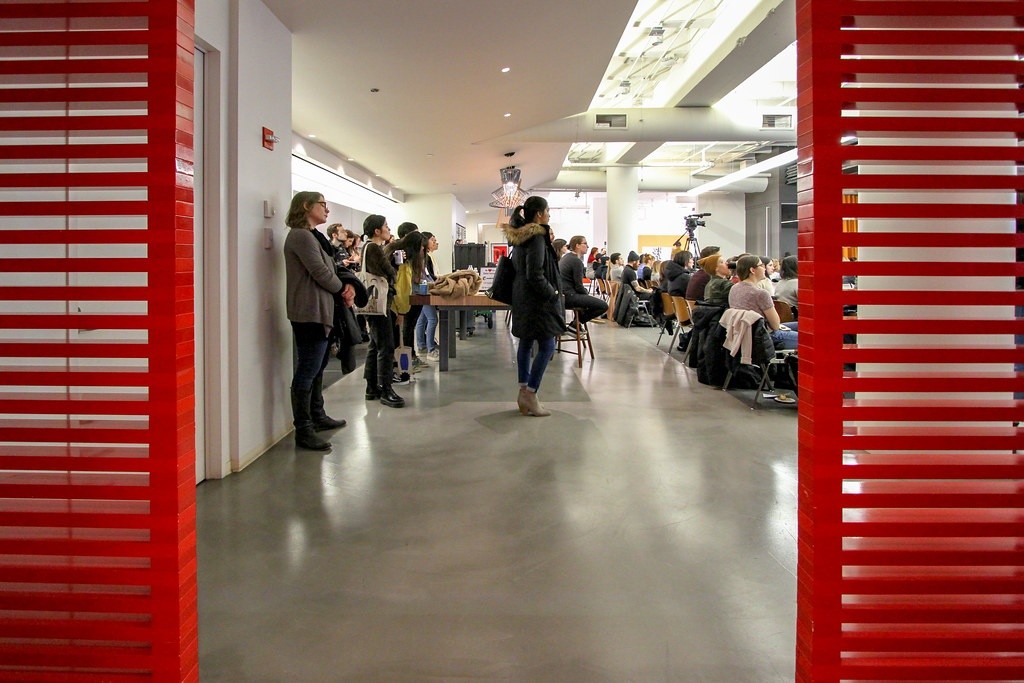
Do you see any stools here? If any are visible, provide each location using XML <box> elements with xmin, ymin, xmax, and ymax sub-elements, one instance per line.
<box><xmin>549</xmin><ymin>307</ymin><xmax>594</xmax><ymax>369</ymax></box>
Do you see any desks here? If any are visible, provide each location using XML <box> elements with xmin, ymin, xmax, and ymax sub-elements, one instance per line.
<box><xmin>408</xmin><ymin>292</ymin><xmax>512</xmax><ymax>373</ymax></box>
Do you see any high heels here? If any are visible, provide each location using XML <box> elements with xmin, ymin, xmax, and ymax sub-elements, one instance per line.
<box><xmin>517</xmin><ymin>388</ymin><xmax>551</xmax><ymax>416</ymax></box>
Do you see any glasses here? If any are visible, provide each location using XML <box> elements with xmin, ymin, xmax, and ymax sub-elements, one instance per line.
<box><xmin>577</xmin><ymin>242</ymin><xmax>587</xmax><ymax>246</ymax></box>
<box><xmin>314</xmin><ymin>201</ymin><xmax>327</xmax><ymax>210</ymax></box>
<box><xmin>755</xmin><ymin>263</ymin><xmax>765</xmax><ymax>269</ymax></box>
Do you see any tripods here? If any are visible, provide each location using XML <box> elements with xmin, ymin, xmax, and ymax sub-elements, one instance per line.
<box><xmin>673</xmin><ymin>226</ymin><xmax>702</xmax><ymax>270</ymax></box>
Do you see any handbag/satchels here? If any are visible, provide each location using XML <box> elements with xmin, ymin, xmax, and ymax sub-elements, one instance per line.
<box><xmin>351</xmin><ymin>242</ymin><xmax>389</xmax><ymax>317</ymax></box>
<box><xmin>486</xmin><ymin>249</ymin><xmax>513</xmax><ymax>304</ymax></box>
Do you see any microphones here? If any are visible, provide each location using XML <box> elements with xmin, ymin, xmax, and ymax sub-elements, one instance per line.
<box><xmin>692</xmin><ymin>213</ymin><xmax>712</xmax><ymax>216</ymax></box>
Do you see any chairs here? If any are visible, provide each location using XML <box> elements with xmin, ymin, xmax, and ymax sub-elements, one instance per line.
<box><xmin>588</xmin><ymin>278</ymin><xmax>798</xmax><ymax>411</ymax></box>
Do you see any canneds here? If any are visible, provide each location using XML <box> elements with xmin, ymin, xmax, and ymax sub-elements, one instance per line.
<box><xmin>394</xmin><ymin>250</ymin><xmax>404</xmax><ymax>264</ymax></box>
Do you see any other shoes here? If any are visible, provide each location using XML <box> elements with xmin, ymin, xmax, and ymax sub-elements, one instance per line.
<box><xmin>416</xmin><ymin>348</ymin><xmax>427</xmax><ymax>356</ymax></box>
<box><xmin>392</xmin><ymin>372</ymin><xmax>410</xmax><ymax>386</ymax></box>
<box><xmin>427</xmin><ymin>349</ymin><xmax>442</xmax><ymax>361</ymax></box>
<box><xmin>412</xmin><ymin>364</ymin><xmax>421</xmax><ymax>373</ymax></box>
<box><xmin>565</xmin><ymin>321</ymin><xmax>587</xmax><ymax>339</ymax></box>
<box><xmin>413</xmin><ymin>356</ymin><xmax>430</xmax><ymax>369</ymax></box>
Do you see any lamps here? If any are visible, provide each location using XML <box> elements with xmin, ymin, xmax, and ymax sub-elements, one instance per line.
<box><xmin>488</xmin><ymin>151</ymin><xmax>530</xmax><ymax>209</ymax></box>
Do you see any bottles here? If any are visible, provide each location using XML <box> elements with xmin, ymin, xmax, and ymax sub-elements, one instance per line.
<box><xmin>468</xmin><ymin>265</ymin><xmax>479</xmax><ymax>275</ymax></box>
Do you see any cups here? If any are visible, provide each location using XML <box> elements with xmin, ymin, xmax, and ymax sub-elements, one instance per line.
<box><xmin>419</xmin><ymin>283</ymin><xmax>428</xmax><ymax>295</ymax></box>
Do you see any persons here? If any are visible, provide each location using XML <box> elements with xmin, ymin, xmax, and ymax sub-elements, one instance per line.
<box><xmin>327</xmin><ymin>214</ymin><xmax>439</xmax><ymax>407</ymax></box>
<box><xmin>501</xmin><ymin>196</ymin><xmax>567</xmax><ymax>416</ymax></box>
<box><xmin>549</xmin><ymin>228</ymin><xmax>609</xmax><ymax>339</ymax></box>
<box><xmin>284</xmin><ymin>191</ymin><xmax>356</xmax><ymax>452</ymax></box>
<box><xmin>586</xmin><ymin>242</ymin><xmax>857</xmax><ymax>392</ymax></box>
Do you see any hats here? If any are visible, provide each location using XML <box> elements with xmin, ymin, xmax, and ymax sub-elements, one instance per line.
<box><xmin>697</xmin><ymin>254</ymin><xmax>721</xmax><ymax>277</ymax></box>
<box><xmin>596</xmin><ymin>252</ymin><xmax>606</xmax><ymax>260</ymax></box>
<box><xmin>628</xmin><ymin>251</ymin><xmax>639</xmax><ymax>262</ymax></box>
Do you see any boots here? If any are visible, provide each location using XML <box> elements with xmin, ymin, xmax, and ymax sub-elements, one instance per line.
<box><xmin>380</xmin><ymin>384</ymin><xmax>405</xmax><ymax>407</ymax></box>
<box><xmin>364</xmin><ymin>377</ymin><xmax>381</xmax><ymax>400</ymax></box>
<box><xmin>289</xmin><ymin>384</ymin><xmax>331</xmax><ymax>450</ymax></box>
<box><xmin>309</xmin><ymin>379</ymin><xmax>346</xmax><ymax>432</ymax></box>
